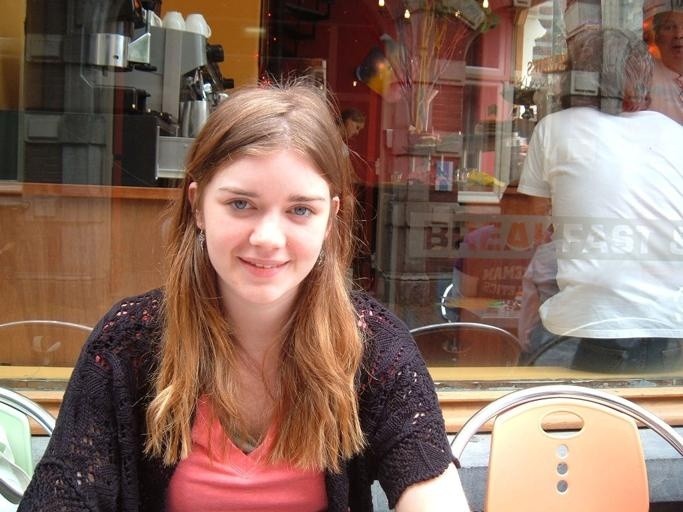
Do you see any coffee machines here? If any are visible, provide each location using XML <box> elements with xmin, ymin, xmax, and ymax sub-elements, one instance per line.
<box><xmin>14</xmin><ymin>0</ymin><xmax>179</xmax><ymax>189</ymax></box>
<box><xmin>112</xmin><ymin>25</ymin><xmax>236</xmax><ymax>184</ymax></box>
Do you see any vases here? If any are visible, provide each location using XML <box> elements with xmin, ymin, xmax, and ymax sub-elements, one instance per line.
<box><xmin>403</xmin><ymin>87</ymin><xmax>440</xmax><ymax>185</ymax></box>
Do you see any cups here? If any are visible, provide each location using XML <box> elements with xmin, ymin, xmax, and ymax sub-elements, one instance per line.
<box><xmin>389</xmin><ymin>168</ymin><xmax>403</xmax><ymax>181</ymax></box>
<box><xmin>144</xmin><ymin>9</ymin><xmax>212</xmax><ymax>40</ymax></box>
<box><xmin>455</xmin><ymin>167</ymin><xmax>480</xmax><ymax>183</ymax></box>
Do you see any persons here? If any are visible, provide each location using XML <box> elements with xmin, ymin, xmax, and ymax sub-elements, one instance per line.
<box><xmin>452</xmin><ymin>177</ymin><xmax>558</xmax><ymax>367</ymax></box>
<box><xmin>337</xmin><ymin>109</ymin><xmax>366</xmax><ymax>141</ymax></box>
<box><xmin>632</xmin><ymin>8</ymin><xmax>682</xmax><ymax>125</ymax></box>
<box><xmin>12</xmin><ymin>70</ymin><xmax>473</xmax><ymax>512</ymax></box>
<box><xmin>516</xmin><ymin>22</ymin><xmax>681</xmax><ymax>375</ymax></box>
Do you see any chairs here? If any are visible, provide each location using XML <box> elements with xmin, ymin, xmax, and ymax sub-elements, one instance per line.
<box><xmin>409</xmin><ymin>282</ymin><xmax>683</xmax><ymax>367</ymax></box>
<box><xmin>0</xmin><ymin>387</ymin><xmax>56</xmax><ymax>512</ymax></box>
<box><xmin>0</xmin><ymin>319</ymin><xmax>93</xmax><ymax>388</ymax></box>
<box><xmin>445</xmin><ymin>385</ymin><xmax>683</xmax><ymax>512</ymax></box>
